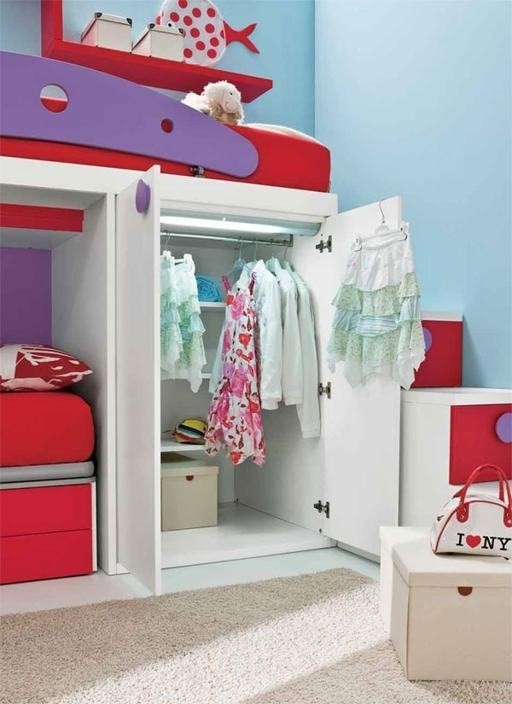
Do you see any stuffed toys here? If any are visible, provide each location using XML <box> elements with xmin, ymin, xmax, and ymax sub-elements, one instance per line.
<box><xmin>184</xmin><ymin>77</ymin><xmax>246</xmax><ymax>127</ymax></box>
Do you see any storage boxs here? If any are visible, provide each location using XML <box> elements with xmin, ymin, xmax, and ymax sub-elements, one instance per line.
<box><xmin>80</xmin><ymin>12</ymin><xmax>132</xmax><ymax>53</ymax></box>
<box><xmin>401</xmin><ymin>313</ymin><xmax>463</xmax><ymax>388</ymax></box>
<box><xmin>132</xmin><ymin>23</ymin><xmax>183</xmax><ymax>62</ymax></box>
<box><xmin>378</xmin><ymin>526</ymin><xmax>512</xmax><ymax>682</ymax></box>
<box><xmin>161</xmin><ymin>453</ymin><xmax>219</xmax><ymax>531</ymax></box>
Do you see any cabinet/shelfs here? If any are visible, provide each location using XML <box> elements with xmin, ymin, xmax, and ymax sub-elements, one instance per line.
<box><xmin>115</xmin><ymin>165</ymin><xmax>401</xmax><ymax>597</ymax></box>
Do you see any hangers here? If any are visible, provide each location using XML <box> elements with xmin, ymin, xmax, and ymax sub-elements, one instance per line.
<box><xmin>220</xmin><ymin>235</ymin><xmax>293</xmax><ymax>281</ymax></box>
<box><xmin>162</xmin><ymin>229</ymin><xmax>184</xmax><ymax>263</ymax></box>
<box><xmin>356</xmin><ymin>198</ymin><xmax>404</xmax><ymax>241</ymax></box>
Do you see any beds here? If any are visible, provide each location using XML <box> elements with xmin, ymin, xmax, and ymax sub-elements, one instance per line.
<box><xmin>0</xmin><ymin>392</ymin><xmax>96</xmax><ymax>586</ymax></box>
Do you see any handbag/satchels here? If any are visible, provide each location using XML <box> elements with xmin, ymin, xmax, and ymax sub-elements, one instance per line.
<box><xmin>430</xmin><ymin>464</ymin><xmax>512</xmax><ymax>560</ymax></box>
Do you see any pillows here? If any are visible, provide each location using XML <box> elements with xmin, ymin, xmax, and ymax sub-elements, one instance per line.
<box><xmin>0</xmin><ymin>344</ymin><xmax>93</xmax><ymax>392</ymax></box>
